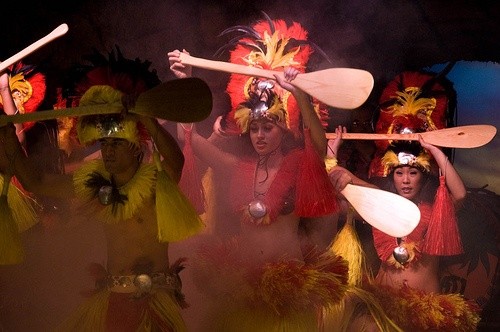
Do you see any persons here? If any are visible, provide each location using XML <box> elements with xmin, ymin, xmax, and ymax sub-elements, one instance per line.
<box><xmin>1</xmin><ymin>64</ymin><xmax>103</xmax><ymax>206</ymax></box>
<box><xmin>166</xmin><ymin>47</ymin><xmax>385</xmax><ymax>332</ymax></box>
<box><xmin>1</xmin><ymin>87</ymin><xmax>204</xmax><ymax>331</ymax></box>
<box><xmin>325</xmin><ymin>116</ymin><xmax>483</xmax><ymax>332</ymax></box>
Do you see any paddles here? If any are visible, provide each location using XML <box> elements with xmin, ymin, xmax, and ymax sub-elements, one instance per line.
<box><xmin>0</xmin><ymin>23</ymin><xmax>69</xmax><ymax>72</ymax></box>
<box><xmin>326</xmin><ymin>124</ymin><xmax>497</xmax><ymax>149</ymax></box>
<box><xmin>176</xmin><ymin>51</ymin><xmax>375</xmax><ymax>111</ymax></box>
<box><xmin>341</xmin><ymin>183</ymin><xmax>421</xmax><ymax>238</ymax></box>
<box><xmin>0</xmin><ymin>77</ymin><xmax>213</xmax><ymax>125</ymax></box>
